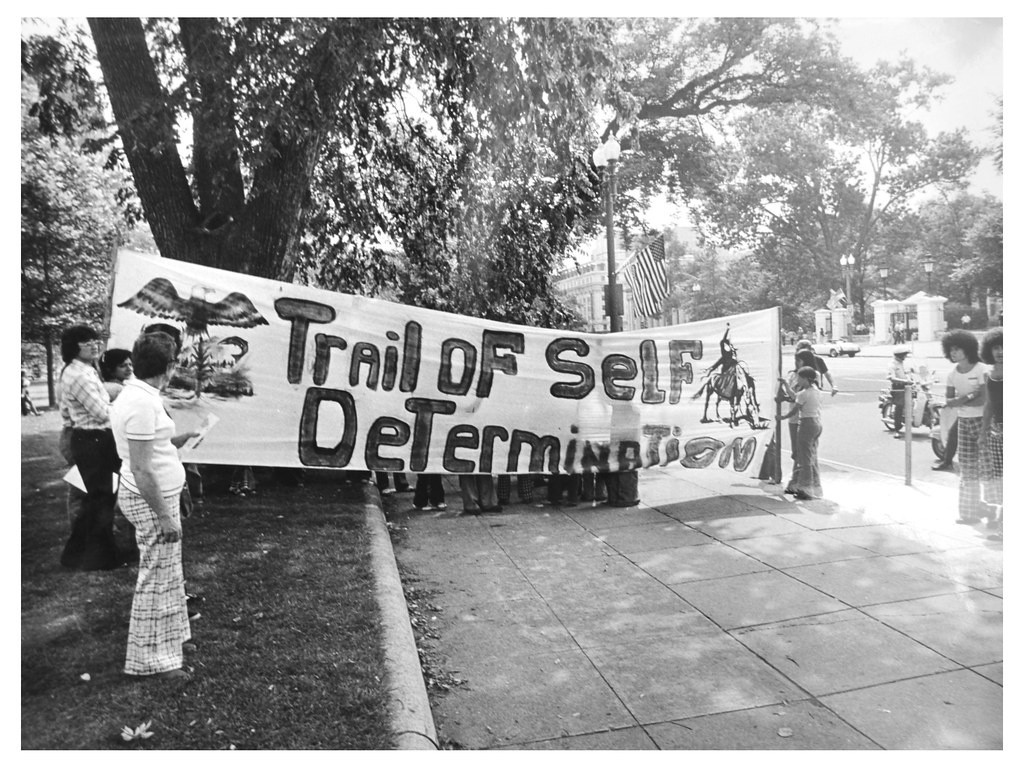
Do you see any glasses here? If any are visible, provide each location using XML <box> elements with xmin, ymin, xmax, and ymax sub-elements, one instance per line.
<box><xmin>79</xmin><ymin>339</ymin><xmax>103</xmax><ymax>348</ymax></box>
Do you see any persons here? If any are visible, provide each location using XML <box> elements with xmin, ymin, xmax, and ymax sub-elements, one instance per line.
<box><xmin>781</xmin><ymin>327</ymin><xmax>803</xmax><ymax>346</ymax></box>
<box><xmin>820</xmin><ymin>328</ymin><xmax>825</xmax><ymax>341</ymax></box>
<box><xmin>889</xmin><ymin>346</ymin><xmax>913</xmax><ymax>440</ymax></box>
<box><xmin>961</xmin><ymin>314</ymin><xmax>970</xmax><ymax>329</ymax></box>
<box><xmin>998</xmin><ymin>310</ymin><xmax>1003</xmax><ymax>327</ymax></box>
<box><xmin>775</xmin><ymin>340</ymin><xmax>837</xmax><ymax>500</ymax></box>
<box><xmin>931</xmin><ymin>328</ymin><xmax>1003</xmax><ymax>541</ymax></box>
<box><xmin>21</xmin><ymin>371</ymin><xmax>42</xmax><ymax>416</ymax></box>
<box><xmin>377</xmin><ymin>470</ymin><xmax>640</xmax><ymax>515</ymax></box>
<box><xmin>884</xmin><ymin>320</ymin><xmax>905</xmax><ymax>345</ymax></box>
<box><xmin>62</xmin><ymin>324</ymin><xmax>201</xmax><ymax>679</ymax></box>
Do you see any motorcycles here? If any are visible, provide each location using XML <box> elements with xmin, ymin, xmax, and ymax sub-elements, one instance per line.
<box><xmin>878</xmin><ymin>368</ymin><xmax>947</xmax><ymax>431</ymax></box>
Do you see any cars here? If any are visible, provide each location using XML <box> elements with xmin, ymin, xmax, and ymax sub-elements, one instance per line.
<box><xmin>810</xmin><ymin>338</ymin><xmax>861</xmax><ymax>358</ymax></box>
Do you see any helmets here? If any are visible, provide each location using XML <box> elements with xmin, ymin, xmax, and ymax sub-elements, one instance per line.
<box><xmin>894</xmin><ymin>344</ymin><xmax>911</xmax><ymax>358</ymax></box>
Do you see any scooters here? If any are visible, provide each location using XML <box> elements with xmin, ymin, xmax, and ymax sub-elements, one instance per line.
<box><xmin>929</xmin><ymin>399</ymin><xmax>960</xmax><ymax>462</ymax></box>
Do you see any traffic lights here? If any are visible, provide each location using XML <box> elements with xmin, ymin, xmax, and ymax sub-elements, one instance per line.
<box><xmin>601</xmin><ymin>285</ymin><xmax>624</xmax><ymax>316</ymax></box>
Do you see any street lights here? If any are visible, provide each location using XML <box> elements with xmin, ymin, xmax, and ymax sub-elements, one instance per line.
<box><xmin>921</xmin><ymin>251</ymin><xmax>936</xmax><ymax>297</ymax></box>
<box><xmin>693</xmin><ymin>282</ymin><xmax>702</xmax><ymax>322</ymax></box>
<box><xmin>840</xmin><ymin>253</ymin><xmax>856</xmax><ymax>341</ymax></box>
<box><xmin>878</xmin><ymin>258</ymin><xmax>891</xmax><ymax>301</ymax></box>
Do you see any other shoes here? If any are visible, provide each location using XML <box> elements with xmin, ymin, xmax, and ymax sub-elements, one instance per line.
<box><xmin>989</xmin><ymin>533</ymin><xmax>1002</xmax><ymax>541</ymax></box>
<box><xmin>793</xmin><ymin>492</ymin><xmax>811</xmax><ymax>499</ymax></box>
<box><xmin>932</xmin><ymin>463</ymin><xmax>951</xmax><ymax>470</ymax></box>
<box><xmin>230</xmin><ymin>478</ymin><xmax>638</xmax><ymax>516</ymax></box>
<box><xmin>189</xmin><ymin>610</ymin><xmax>199</xmax><ymax>623</ymax></box>
<box><xmin>785</xmin><ymin>488</ymin><xmax>794</xmax><ymax>494</ymax></box>
<box><xmin>895</xmin><ymin>430</ymin><xmax>901</xmax><ymax>438</ymax></box>
<box><xmin>957</xmin><ymin>515</ymin><xmax>980</xmax><ymax>525</ymax></box>
<box><xmin>154</xmin><ymin>669</ymin><xmax>191</xmax><ymax>681</ymax></box>
<box><xmin>183</xmin><ymin>643</ymin><xmax>196</xmax><ymax>654</ymax></box>
